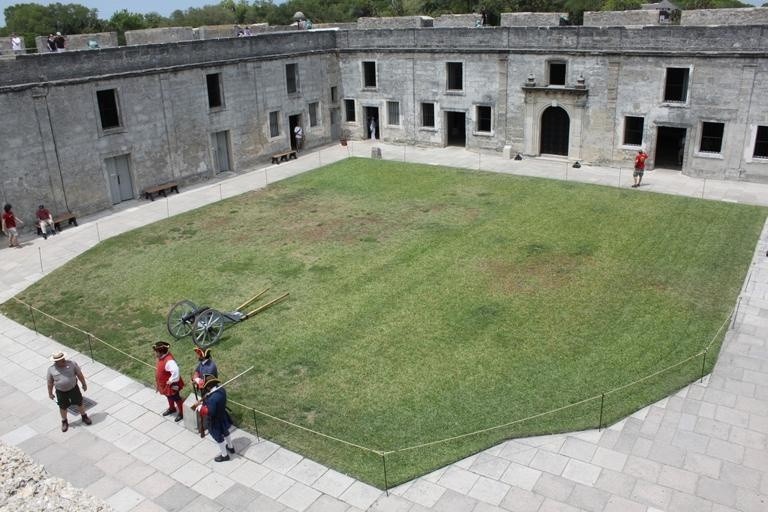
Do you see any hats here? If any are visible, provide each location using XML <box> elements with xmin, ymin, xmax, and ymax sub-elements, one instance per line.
<box><xmin>151</xmin><ymin>341</ymin><xmax>169</xmax><ymax>353</ymax></box>
<box><xmin>193</xmin><ymin>348</ymin><xmax>212</xmax><ymax>358</ymax></box>
<box><xmin>203</xmin><ymin>373</ymin><xmax>221</xmax><ymax>388</ymax></box>
<box><xmin>50</xmin><ymin>351</ymin><xmax>65</xmax><ymax>362</ymax></box>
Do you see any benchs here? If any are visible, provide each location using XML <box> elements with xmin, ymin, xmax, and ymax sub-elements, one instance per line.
<box><xmin>145</xmin><ymin>183</ymin><xmax>179</xmax><ymax>201</ymax></box>
<box><xmin>33</xmin><ymin>214</ymin><xmax>78</xmax><ymax>237</ymax></box>
<box><xmin>271</xmin><ymin>151</ymin><xmax>297</xmax><ymax>164</ymax></box>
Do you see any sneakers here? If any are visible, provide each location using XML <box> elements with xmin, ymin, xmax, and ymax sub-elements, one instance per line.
<box><xmin>51</xmin><ymin>230</ymin><xmax>55</xmax><ymax>234</ymax></box>
<box><xmin>62</xmin><ymin>420</ymin><xmax>68</xmax><ymax>432</ymax></box>
<box><xmin>163</xmin><ymin>409</ymin><xmax>176</xmax><ymax>416</ymax></box>
<box><xmin>175</xmin><ymin>415</ymin><xmax>183</xmax><ymax>421</ymax></box>
<box><xmin>632</xmin><ymin>184</ymin><xmax>640</xmax><ymax>187</ymax></box>
<box><xmin>81</xmin><ymin>414</ymin><xmax>91</xmax><ymax>424</ymax></box>
<box><xmin>43</xmin><ymin>233</ymin><xmax>47</xmax><ymax>239</ymax></box>
<box><xmin>9</xmin><ymin>244</ymin><xmax>22</xmax><ymax>248</ymax></box>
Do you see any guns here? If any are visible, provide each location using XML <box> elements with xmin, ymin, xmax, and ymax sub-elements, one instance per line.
<box><xmin>192</xmin><ymin>379</ymin><xmax>206</xmax><ymax>438</ymax></box>
<box><xmin>191</xmin><ymin>365</ymin><xmax>253</xmax><ymax>412</ymax></box>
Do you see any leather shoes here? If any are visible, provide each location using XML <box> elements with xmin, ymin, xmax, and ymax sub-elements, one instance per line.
<box><xmin>226</xmin><ymin>444</ymin><xmax>234</xmax><ymax>453</ymax></box>
<box><xmin>215</xmin><ymin>454</ymin><xmax>229</xmax><ymax>462</ymax></box>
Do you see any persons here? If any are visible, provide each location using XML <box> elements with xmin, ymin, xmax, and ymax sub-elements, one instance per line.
<box><xmin>369</xmin><ymin>116</ymin><xmax>377</xmax><ymax>141</ymax></box>
<box><xmin>631</xmin><ymin>149</ymin><xmax>648</xmax><ymax>186</ymax></box>
<box><xmin>244</xmin><ymin>26</ymin><xmax>251</xmax><ymax>36</ymax></box>
<box><xmin>657</xmin><ymin>7</ymin><xmax>680</xmax><ymax>24</ymax></box>
<box><xmin>234</xmin><ymin>22</ymin><xmax>244</xmax><ymax>38</ymax></box>
<box><xmin>480</xmin><ymin>12</ymin><xmax>487</xmax><ymax>27</ymax></box>
<box><xmin>677</xmin><ymin>137</ymin><xmax>685</xmax><ymax>165</ymax></box>
<box><xmin>200</xmin><ymin>372</ymin><xmax>235</xmax><ymax>462</ymax></box>
<box><xmin>2</xmin><ymin>202</ymin><xmax>25</xmax><ymax>248</ymax></box>
<box><xmin>10</xmin><ymin>32</ymin><xmax>21</xmax><ymax>56</ymax></box>
<box><xmin>48</xmin><ymin>350</ymin><xmax>91</xmax><ymax>432</ymax></box>
<box><xmin>36</xmin><ymin>204</ymin><xmax>56</xmax><ymax>239</ymax></box>
<box><xmin>293</xmin><ymin>125</ymin><xmax>304</xmax><ymax>153</ymax></box>
<box><xmin>190</xmin><ymin>347</ymin><xmax>216</xmax><ymax>407</ymax></box>
<box><xmin>54</xmin><ymin>32</ymin><xmax>65</xmax><ymax>52</ymax></box>
<box><xmin>290</xmin><ymin>19</ymin><xmax>312</xmax><ymax>30</ymax></box>
<box><xmin>153</xmin><ymin>342</ymin><xmax>185</xmax><ymax>422</ymax></box>
<box><xmin>47</xmin><ymin>34</ymin><xmax>56</xmax><ymax>53</ymax></box>
